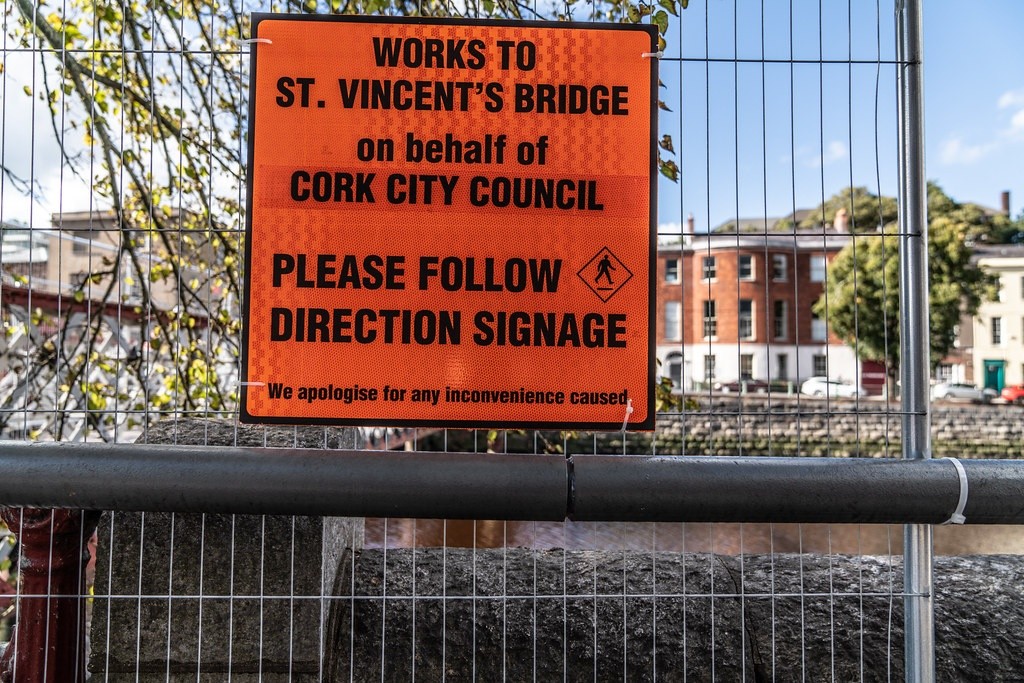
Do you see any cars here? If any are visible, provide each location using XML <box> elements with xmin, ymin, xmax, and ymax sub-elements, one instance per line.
<box><xmin>717</xmin><ymin>378</ymin><xmax>771</xmax><ymax>394</ymax></box>
<box><xmin>801</xmin><ymin>377</ymin><xmax>866</xmax><ymax>400</ymax></box>
<box><xmin>1002</xmin><ymin>385</ymin><xmax>1024</xmax><ymax>403</ymax></box>
<box><xmin>932</xmin><ymin>380</ymin><xmax>998</xmax><ymax>402</ymax></box>
